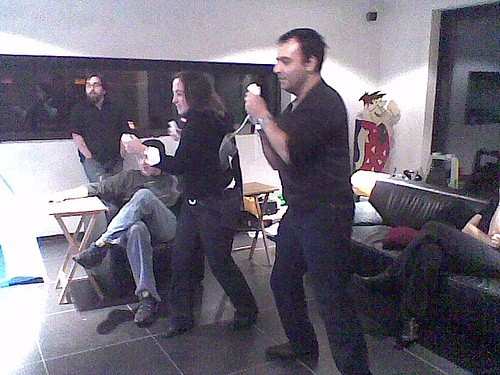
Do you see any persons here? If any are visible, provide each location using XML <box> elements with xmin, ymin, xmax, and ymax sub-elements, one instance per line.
<box><xmin>120</xmin><ymin>69</ymin><xmax>259</xmax><ymax>338</ymax></box>
<box><xmin>50</xmin><ymin>149</ymin><xmax>181</xmax><ymax>327</ymax></box>
<box><xmin>241</xmin><ymin>28</ymin><xmax>373</xmax><ymax>375</ymax></box>
<box><xmin>350</xmin><ymin>196</ymin><xmax>500</xmax><ymax>348</ymax></box>
<box><xmin>70</xmin><ymin>73</ymin><xmax>131</xmax><ymax>222</ymax></box>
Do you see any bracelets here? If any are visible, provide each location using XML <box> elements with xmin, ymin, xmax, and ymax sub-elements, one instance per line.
<box><xmin>259</xmin><ymin>114</ymin><xmax>273</xmax><ymax>127</ymax></box>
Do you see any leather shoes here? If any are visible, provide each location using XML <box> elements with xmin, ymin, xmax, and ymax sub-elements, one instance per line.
<box><xmin>265</xmin><ymin>342</ymin><xmax>318</xmax><ymax>358</ymax></box>
<box><xmin>72</xmin><ymin>243</ymin><xmax>107</xmax><ymax>267</ymax></box>
<box><xmin>163</xmin><ymin>318</ymin><xmax>194</xmax><ymax>339</ymax></box>
<box><xmin>397</xmin><ymin>316</ymin><xmax>420</xmax><ymax>350</ymax></box>
<box><xmin>134</xmin><ymin>290</ymin><xmax>160</xmax><ymax>325</ymax></box>
<box><xmin>352</xmin><ymin>268</ymin><xmax>396</xmax><ymax>295</ymax></box>
<box><xmin>228</xmin><ymin>313</ymin><xmax>257</xmax><ymax>331</ymax></box>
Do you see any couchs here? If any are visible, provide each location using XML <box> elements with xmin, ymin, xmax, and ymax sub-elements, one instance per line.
<box><xmin>341</xmin><ymin>178</ymin><xmax>499</xmax><ymax>353</ymax></box>
<box><xmin>104</xmin><ymin>194</ymin><xmax>176</xmax><ymax>299</ymax></box>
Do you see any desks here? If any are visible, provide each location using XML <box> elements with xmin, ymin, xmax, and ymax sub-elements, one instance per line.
<box><xmin>243</xmin><ymin>181</ymin><xmax>280</xmax><ymax>266</ymax></box>
<box><xmin>47</xmin><ymin>195</ymin><xmax>111</xmax><ymax>307</ymax></box>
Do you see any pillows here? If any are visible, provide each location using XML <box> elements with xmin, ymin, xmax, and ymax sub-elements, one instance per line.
<box><xmin>379</xmin><ymin>226</ymin><xmax>419</xmax><ymax>246</ymax></box>
